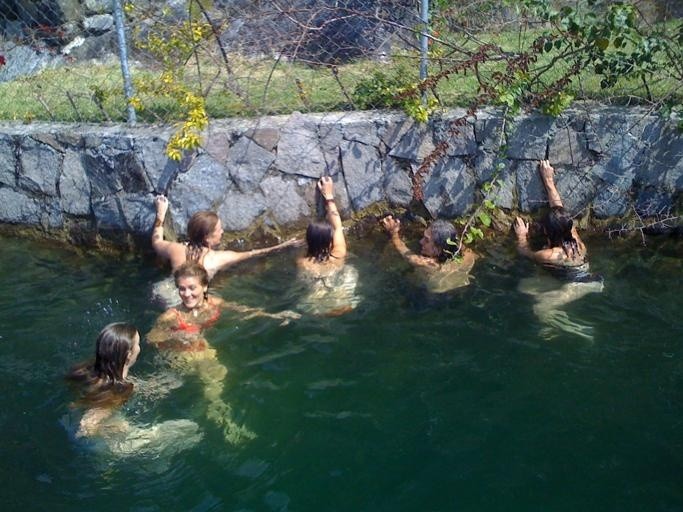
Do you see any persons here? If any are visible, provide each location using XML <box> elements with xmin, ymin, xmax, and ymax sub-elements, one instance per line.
<box><xmin>294</xmin><ymin>175</ymin><xmax>365</xmax><ymax>318</ymax></box>
<box><xmin>379</xmin><ymin>212</ymin><xmax>478</xmax><ymax>296</ymax></box>
<box><xmin>511</xmin><ymin>159</ymin><xmax>605</xmax><ymax>345</ymax></box>
<box><xmin>143</xmin><ymin>261</ymin><xmax>302</xmax><ymax>445</ymax></box>
<box><xmin>65</xmin><ymin>319</ymin><xmax>205</xmax><ymax>460</ymax></box>
<box><xmin>149</xmin><ymin>195</ymin><xmax>305</xmax><ymax>309</ymax></box>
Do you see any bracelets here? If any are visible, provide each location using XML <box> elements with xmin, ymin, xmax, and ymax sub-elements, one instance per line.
<box><xmin>153</xmin><ymin>218</ymin><xmax>164</xmax><ymax>227</ymax></box>
<box><xmin>324</xmin><ymin>199</ymin><xmax>335</xmax><ymax>205</ymax></box>
